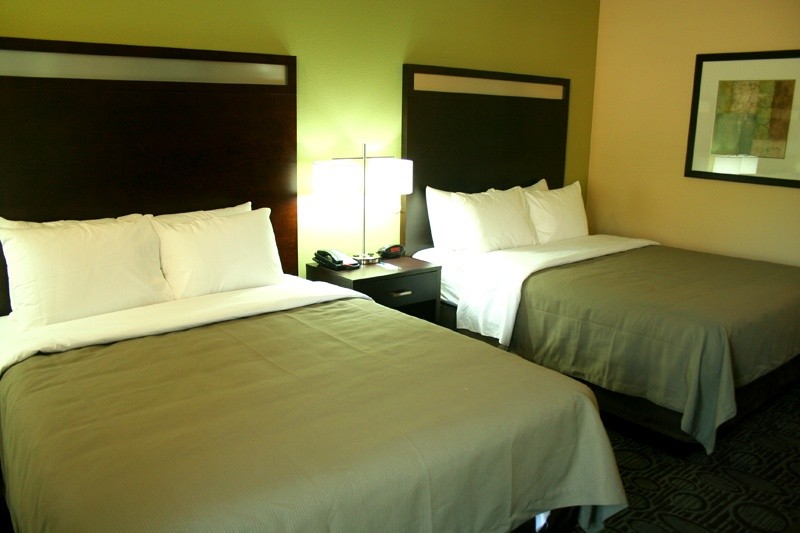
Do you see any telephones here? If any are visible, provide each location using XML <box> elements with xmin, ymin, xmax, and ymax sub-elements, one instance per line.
<box><xmin>313</xmin><ymin>250</ymin><xmax>359</xmax><ymax>271</ymax></box>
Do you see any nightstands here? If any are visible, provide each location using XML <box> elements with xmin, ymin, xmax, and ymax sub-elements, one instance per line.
<box><xmin>306</xmin><ymin>256</ymin><xmax>442</xmax><ymax>323</ymax></box>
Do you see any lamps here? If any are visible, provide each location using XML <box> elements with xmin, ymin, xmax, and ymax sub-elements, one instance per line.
<box><xmin>313</xmin><ymin>145</ymin><xmax>414</xmax><ymax>264</ymax></box>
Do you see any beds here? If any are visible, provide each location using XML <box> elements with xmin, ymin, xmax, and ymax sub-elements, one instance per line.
<box><xmin>0</xmin><ymin>37</ymin><xmax>623</xmax><ymax>533</ymax></box>
<box><xmin>402</xmin><ymin>64</ymin><xmax>800</xmax><ymax>456</ymax></box>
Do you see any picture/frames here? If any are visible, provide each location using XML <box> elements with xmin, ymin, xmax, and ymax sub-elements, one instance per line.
<box><xmin>684</xmin><ymin>49</ymin><xmax>800</xmax><ymax>188</ymax></box>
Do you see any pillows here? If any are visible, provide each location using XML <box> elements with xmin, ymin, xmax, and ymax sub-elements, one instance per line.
<box><xmin>426</xmin><ymin>179</ymin><xmax>588</xmax><ymax>251</ymax></box>
<box><xmin>0</xmin><ymin>201</ymin><xmax>285</xmax><ymax>326</ymax></box>
